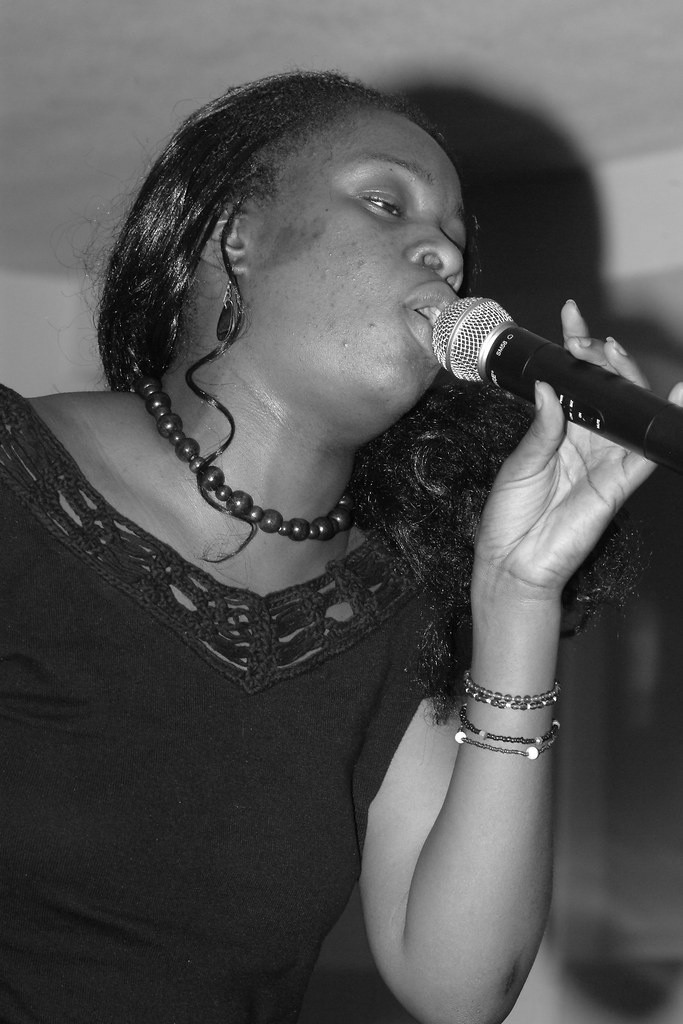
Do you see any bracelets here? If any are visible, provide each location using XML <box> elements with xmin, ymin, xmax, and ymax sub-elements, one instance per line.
<box><xmin>464</xmin><ymin>672</ymin><xmax>563</xmax><ymax>711</ymax></box>
<box><xmin>455</xmin><ymin>709</ymin><xmax>559</xmax><ymax>758</ymax></box>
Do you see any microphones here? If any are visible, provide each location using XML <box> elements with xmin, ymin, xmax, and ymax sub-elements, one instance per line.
<box><xmin>433</xmin><ymin>297</ymin><xmax>683</xmax><ymax>475</ymax></box>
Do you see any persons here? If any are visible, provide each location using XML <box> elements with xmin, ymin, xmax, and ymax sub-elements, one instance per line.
<box><xmin>2</xmin><ymin>70</ymin><xmax>682</xmax><ymax>1024</ymax></box>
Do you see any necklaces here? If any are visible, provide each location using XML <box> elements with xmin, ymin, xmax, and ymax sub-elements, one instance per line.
<box><xmin>136</xmin><ymin>375</ymin><xmax>358</xmax><ymax>541</ymax></box>
